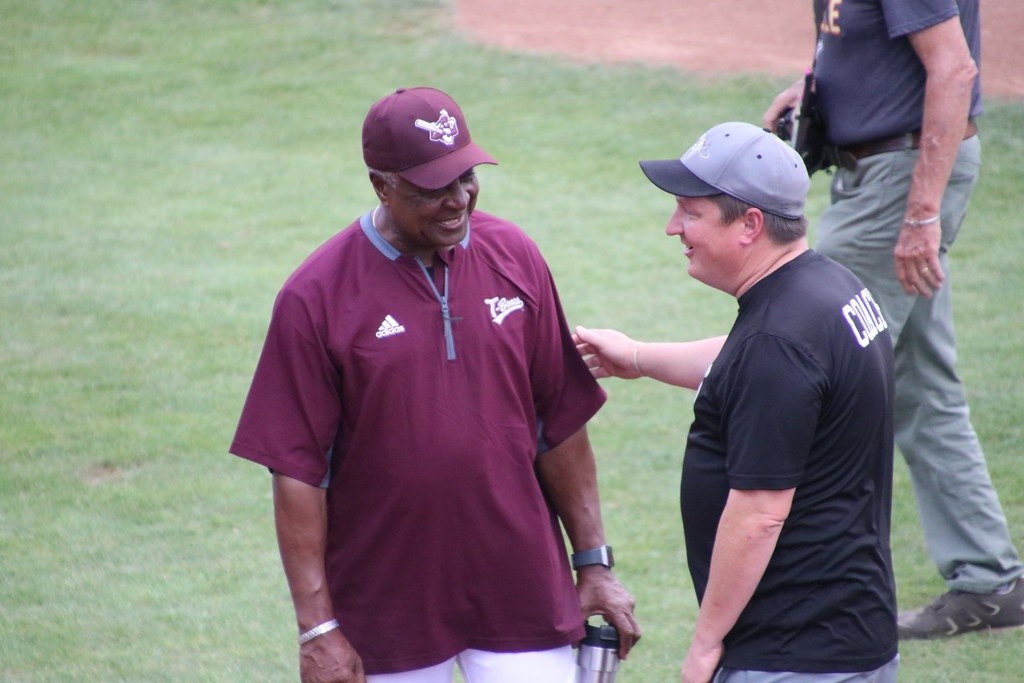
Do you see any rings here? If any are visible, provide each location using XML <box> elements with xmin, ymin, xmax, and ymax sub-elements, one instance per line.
<box><xmin>919</xmin><ymin>267</ymin><xmax>929</xmax><ymax>272</ymax></box>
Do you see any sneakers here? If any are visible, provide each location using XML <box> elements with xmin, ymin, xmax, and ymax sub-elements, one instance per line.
<box><xmin>896</xmin><ymin>576</ymin><xmax>1024</xmax><ymax>637</ymax></box>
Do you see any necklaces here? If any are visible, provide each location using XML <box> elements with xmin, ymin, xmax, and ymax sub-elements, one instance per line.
<box><xmin>373</xmin><ymin>204</ymin><xmax>379</xmax><ymax>228</ymax></box>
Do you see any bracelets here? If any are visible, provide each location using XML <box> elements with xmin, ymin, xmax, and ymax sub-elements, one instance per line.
<box><xmin>903</xmin><ymin>216</ymin><xmax>937</xmax><ymax>225</ymax></box>
<box><xmin>297</xmin><ymin>619</ymin><xmax>338</xmax><ymax>646</ymax></box>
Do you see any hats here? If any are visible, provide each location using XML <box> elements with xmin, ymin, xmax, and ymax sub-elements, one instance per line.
<box><xmin>639</xmin><ymin>121</ymin><xmax>810</xmax><ymax>220</ymax></box>
<box><xmin>361</xmin><ymin>86</ymin><xmax>498</xmax><ymax>189</ymax></box>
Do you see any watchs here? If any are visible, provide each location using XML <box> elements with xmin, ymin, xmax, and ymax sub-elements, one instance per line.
<box><xmin>571</xmin><ymin>545</ymin><xmax>615</xmax><ymax>570</ymax></box>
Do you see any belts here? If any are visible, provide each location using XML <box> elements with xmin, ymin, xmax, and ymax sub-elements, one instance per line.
<box><xmin>841</xmin><ymin>118</ymin><xmax>978</xmax><ymax>169</ymax></box>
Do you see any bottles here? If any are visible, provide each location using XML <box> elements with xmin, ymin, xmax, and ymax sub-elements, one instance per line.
<box><xmin>574</xmin><ymin>618</ymin><xmax>620</xmax><ymax>683</ymax></box>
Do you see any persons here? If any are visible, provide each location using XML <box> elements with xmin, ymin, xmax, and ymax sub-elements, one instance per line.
<box><xmin>765</xmin><ymin>1</ymin><xmax>1024</xmax><ymax>637</ymax></box>
<box><xmin>230</xmin><ymin>86</ymin><xmax>641</xmax><ymax>683</ymax></box>
<box><xmin>572</xmin><ymin>120</ymin><xmax>902</xmax><ymax>683</ymax></box>
